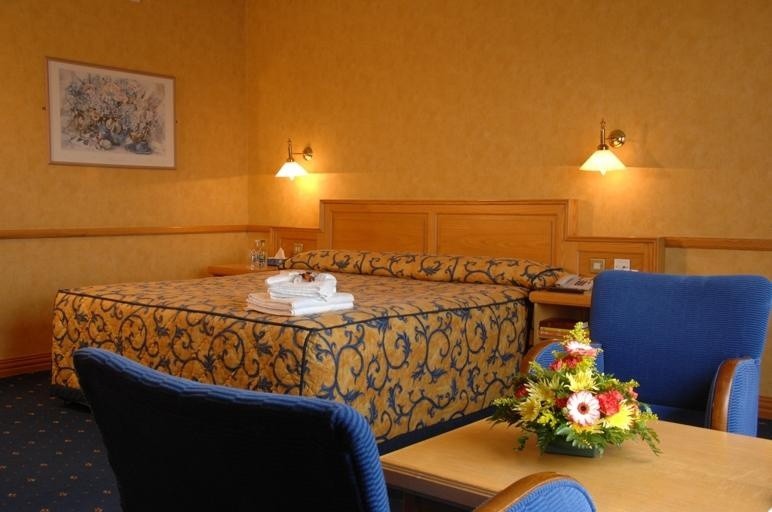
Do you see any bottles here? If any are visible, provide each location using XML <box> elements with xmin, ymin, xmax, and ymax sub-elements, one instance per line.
<box><xmin>249</xmin><ymin>239</ymin><xmax>268</xmax><ymax>271</ymax></box>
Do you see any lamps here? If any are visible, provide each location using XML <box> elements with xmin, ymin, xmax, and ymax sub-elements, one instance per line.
<box><xmin>271</xmin><ymin>137</ymin><xmax>313</xmax><ymax>181</ymax></box>
<box><xmin>579</xmin><ymin>118</ymin><xmax>629</xmax><ymax>178</ymax></box>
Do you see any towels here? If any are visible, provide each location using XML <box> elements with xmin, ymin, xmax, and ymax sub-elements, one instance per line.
<box><xmin>264</xmin><ymin>266</ymin><xmax>338</xmax><ymax>301</ymax></box>
<box><xmin>241</xmin><ymin>291</ymin><xmax>355</xmax><ymax>317</ymax></box>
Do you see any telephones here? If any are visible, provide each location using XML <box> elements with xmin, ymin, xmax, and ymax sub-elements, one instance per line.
<box><xmin>555</xmin><ymin>274</ymin><xmax>594</xmax><ymax>291</ymax></box>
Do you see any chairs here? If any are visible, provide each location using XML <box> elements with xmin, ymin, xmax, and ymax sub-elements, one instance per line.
<box><xmin>73</xmin><ymin>346</ymin><xmax>599</xmax><ymax>512</ymax></box>
<box><xmin>516</xmin><ymin>268</ymin><xmax>771</xmax><ymax>437</ymax></box>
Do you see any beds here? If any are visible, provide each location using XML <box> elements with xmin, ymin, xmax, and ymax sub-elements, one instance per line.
<box><xmin>49</xmin><ymin>199</ymin><xmax>578</xmax><ymax>445</ymax></box>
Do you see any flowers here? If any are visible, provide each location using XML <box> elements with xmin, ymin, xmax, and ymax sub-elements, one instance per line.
<box><xmin>486</xmin><ymin>318</ymin><xmax>664</xmax><ymax>459</ymax></box>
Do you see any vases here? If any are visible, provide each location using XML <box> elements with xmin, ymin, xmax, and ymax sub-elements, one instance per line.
<box><xmin>535</xmin><ymin>429</ymin><xmax>604</xmax><ymax>459</ymax></box>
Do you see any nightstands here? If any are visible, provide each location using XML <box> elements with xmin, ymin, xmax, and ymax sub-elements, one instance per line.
<box><xmin>207</xmin><ymin>263</ymin><xmax>273</xmax><ymax>277</ymax></box>
<box><xmin>526</xmin><ymin>290</ymin><xmax>592</xmax><ymax>347</ymax></box>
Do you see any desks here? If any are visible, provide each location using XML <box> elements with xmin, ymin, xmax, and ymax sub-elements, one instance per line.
<box><xmin>379</xmin><ymin>400</ymin><xmax>772</xmax><ymax>511</ymax></box>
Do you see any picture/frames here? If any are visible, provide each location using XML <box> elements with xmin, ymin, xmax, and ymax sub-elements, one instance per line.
<box><xmin>42</xmin><ymin>56</ymin><xmax>178</xmax><ymax>172</ymax></box>
